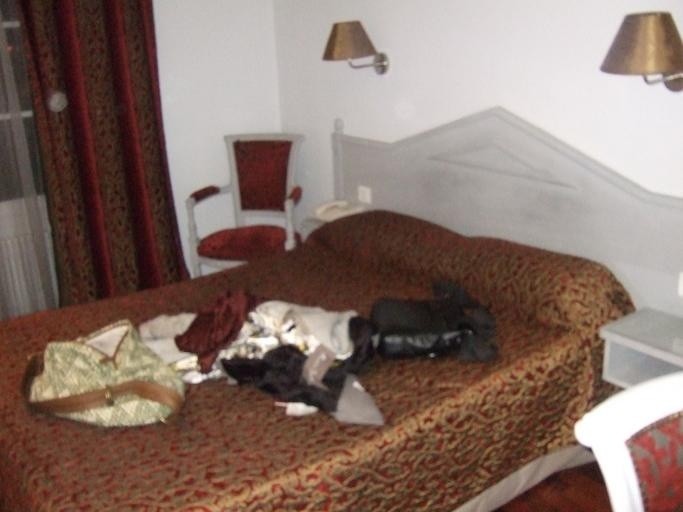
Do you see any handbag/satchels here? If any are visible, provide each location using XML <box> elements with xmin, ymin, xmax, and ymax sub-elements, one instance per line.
<box><xmin>372</xmin><ymin>296</ymin><xmax>474</xmax><ymax>358</ymax></box>
<box><xmin>22</xmin><ymin>317</ymin><xmax>186</xmax><ymax>428</ymax></box>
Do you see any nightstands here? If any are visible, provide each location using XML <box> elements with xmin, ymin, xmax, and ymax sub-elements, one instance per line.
<box><xmin>595</xmin><ymin>307</ymin><xmax>683</xmax><ymax>390</ymax></box>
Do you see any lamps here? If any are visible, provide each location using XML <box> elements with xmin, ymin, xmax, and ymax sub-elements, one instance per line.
<box><xmin>598</xmin><ymin>12</ymin><xmax>682</xmax><ymax>91</ymax></box>
<box><xmin>319</xmin><ymin>20</ymin><xmax>388</xmax><ymax>75</ymax></box>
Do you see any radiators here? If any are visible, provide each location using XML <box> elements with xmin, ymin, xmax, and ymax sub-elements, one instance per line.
<box><xmin>0</xmin><ymin>231</ymin><xmax>62</xmax><ymax>318</ymax></box>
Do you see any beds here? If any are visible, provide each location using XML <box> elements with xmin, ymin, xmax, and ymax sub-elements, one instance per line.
<box><xmin>0</xmin><ymin>104</ymin><xmax>683</xmax><ymax>511</ymax></box>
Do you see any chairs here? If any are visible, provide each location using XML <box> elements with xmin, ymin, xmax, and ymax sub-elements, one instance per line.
<box><xmin>185</xmin><ymin>132</ymin><xmax>301</xmax><ymax>277</ymax></box>
<box><xmin>574</xmin><ymin>370</ymin><xmax>683</xmax><ymax>512</ymax></box>
